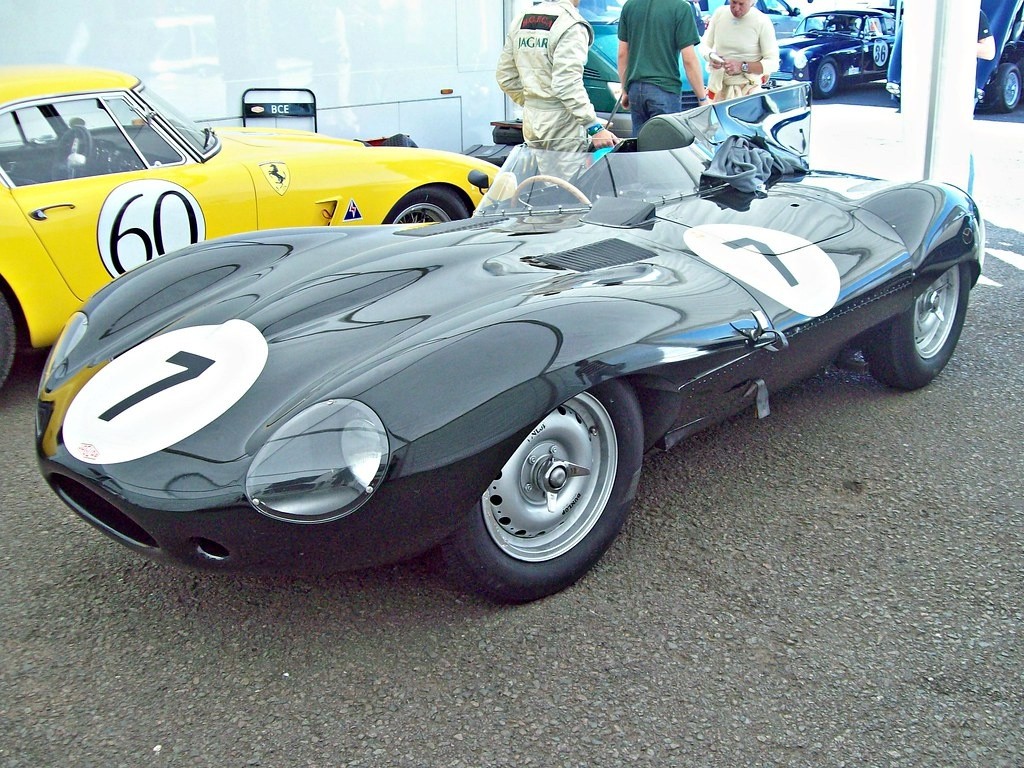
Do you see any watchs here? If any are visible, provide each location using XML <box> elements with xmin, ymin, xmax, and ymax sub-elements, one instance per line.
<box><xmin>741</xmin><ymin>61</ymin><xmax>749</xmax><ymax>73</ymax></box>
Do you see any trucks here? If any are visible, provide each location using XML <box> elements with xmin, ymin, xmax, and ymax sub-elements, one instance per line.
<box><xmin>573</xmin><ymin>0</ymin><xmax>716</xmax><ymax>139</ymax></box>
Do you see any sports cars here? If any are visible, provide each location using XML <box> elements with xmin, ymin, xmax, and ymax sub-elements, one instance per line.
<box><xmin>32</xmin><ymin>146</ymin><xmax>986</xmax><ymax>606</ymax></box>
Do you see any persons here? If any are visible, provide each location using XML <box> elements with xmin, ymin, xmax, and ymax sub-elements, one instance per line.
<box><xmin>698</xmin><ymin>0</ymin><xmax>780</xmax><ymax>104</ymax></box>
<box><xmin>617</xmin><ymin>0</ymin><xmax>709</xmax><ymax>138</ymax></box>
<box><xmin>496</xmin><ymin>0</ymin><xmax>620</xmax><ymax>187</ymax></box>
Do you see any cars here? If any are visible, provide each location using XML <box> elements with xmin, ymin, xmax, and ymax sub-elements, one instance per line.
<box><xmin>0</xmin><ymin>66</ymin><xmax>519</xmax><ymax>388</ymax></box>
<box><xmin>775</xmin><ymin>9</ymin><xmax>897</xmax><ymax>99</ymax></box>
<box><xmin>697</xmin><ymin>0</ymin><xmax>825</xmax><ymax>38</ymax></box>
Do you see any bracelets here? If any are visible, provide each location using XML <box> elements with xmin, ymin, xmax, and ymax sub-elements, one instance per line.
<box><xmin>698</xmin><ymin>97</ymin><xmax>707</xmax><ymax>102</ymax></box>
<box><xmin>587</xmin><ymin>123</ymin><xmax>605</xmax><ymax>137</ymax></box>
